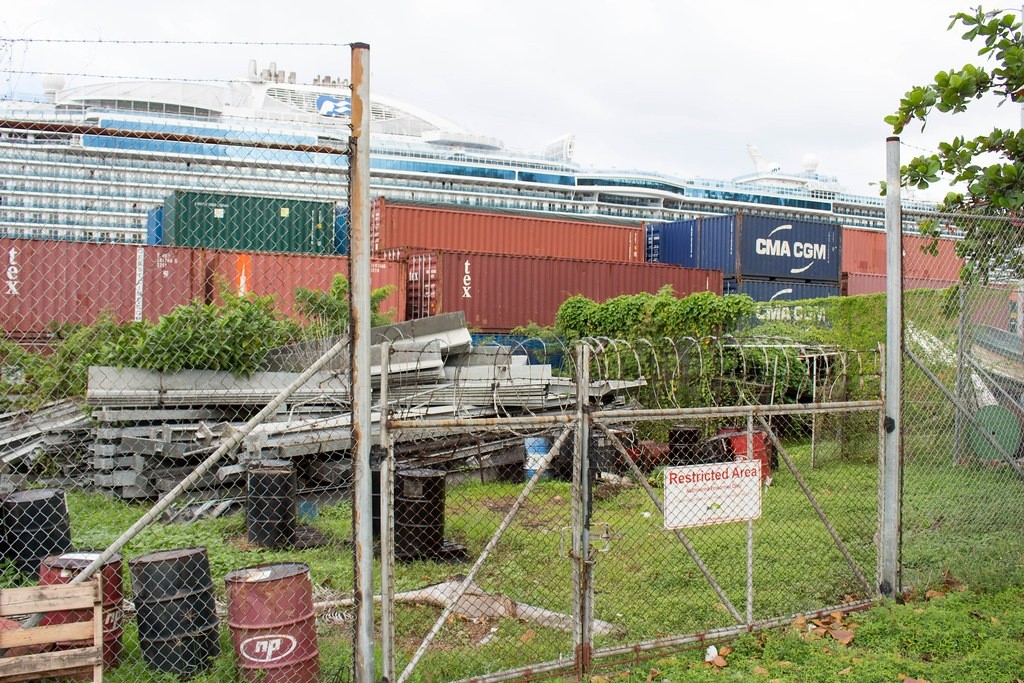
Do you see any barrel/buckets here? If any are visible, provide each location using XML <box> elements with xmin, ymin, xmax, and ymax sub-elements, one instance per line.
<box><xmin>559</xmin><ymin>428</ymin><xmax>572</xmax><ymax>482</ymax></box>
<box><xmin>225</xmin><ymin>562</ymin><xmax>319</xmax><ymax>683</ymax></box>
<box><xmin>590</xmin><ymin>436</ymin><xmax>616</xmax><ymax>479</ymax></box>
<box><xmin>732</xmin><ymin>430</ymin><xmax>770</xmax><ymax>480</ymax></box>
<box><xmin>669</xmin><ymin>430</ymin><xmax>698</xmax><ymax>464</ymax></box>
<box><xmin>523</xmin><ymin>438</ymin><xmax>554</xmax><ymax>481</ymax></box>
<box><xmin>127</xmin><ymin>546</ymin><xmax>222</xmax><ymax>683</ymax></box>
<box><xmin>397</xmin><ymin>470</ymin><xmax>446</xmax><ymax>557</ymax></box>
<box><xmin>614</xmin><ymin>431</ymin><xmax>638</xmax><ymax>470</ymax></box>
<box><xmin>693</xmin><ymin>437</ymin><xmax>733</xmax><ymax>463</ymax></box>
<box><xmin>246</xmin><ymin>460</ymin><xmax>293</xmax><ymax>475</ymax></box>
<box><xmin>718</xmin><ymin>427</ymin><xmax>740</xmax><ymax>435</ymax></box>
<box><xmin>39</xmin><ymin>551</ymin><xmax>122</xmax><ymax>679</ymax></box>
<box><xmin>4</xmin><ymin>487</ymin><xmax>72</xmax><ymax>578</ymax></box>
<box><xmin>369</xmin><ymin>465</ymin><xmax>409</xmax><ymax>547</ymax></box>
<box><xmin>248</xmin><ymin>468</ymin><xmax>295</xmax><ymax>551</ymax></box>
<box><xmin>753</xmin><ymin>427</ymin><xmax>778</xmax><ymax>472</ymax></box>
